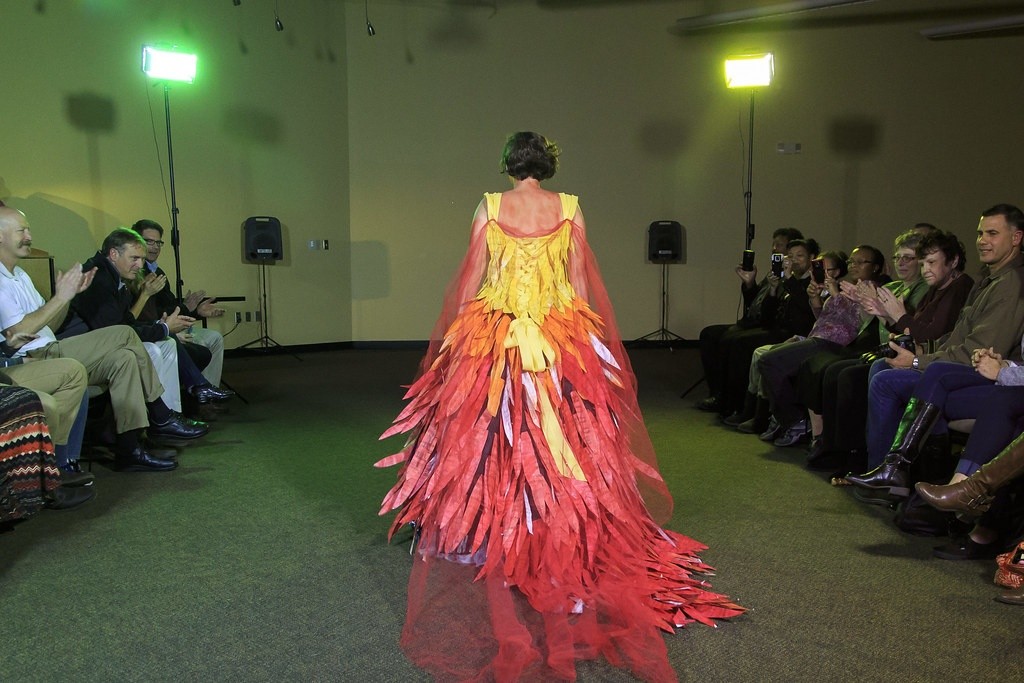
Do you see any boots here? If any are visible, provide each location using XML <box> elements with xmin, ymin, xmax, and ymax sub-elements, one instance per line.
<box><xmin>844</xmin><ymin>394</ymin><xmax>944</xmax><ymax>496</ymax></box>
<box><xmin>914</xmin><ymin>432</ymin><xmax>1024</xmax><ymax>524</ymax></box>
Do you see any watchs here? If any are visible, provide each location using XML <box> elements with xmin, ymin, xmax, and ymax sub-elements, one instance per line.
<box><xmin>911</xmin><ymin>355</ymin><xmax>919</xmax><ymax>369</ymax></box>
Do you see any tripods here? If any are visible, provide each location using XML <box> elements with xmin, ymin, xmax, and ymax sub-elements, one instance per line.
<box><xmin>226</xmin><ymin>264</ymin><xmax>304</xmax><ymax>362</ymax></box>
<box><xmin>622</xmin><ymin>264</ymin><xmax>698</xmax><ymax>352</ymax></box>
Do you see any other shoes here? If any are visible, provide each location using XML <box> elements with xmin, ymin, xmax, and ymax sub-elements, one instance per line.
<box><xmin>828</xmin><ymin>448</ymin><xmax>864</xmax><ymax>486</ymax></box>
<box><xmin>808</xmin><ymin>452</ymin><xmax>845</xmax><ymax>468</ymax></box>
<box><xmin>933</xmin><ymin>535</ymin><xmax>1006</xmax><ymax>560</ymax></box>
<box><xmin>696</xmin><ymin>393</ymin><xmax>723</xmax><ymax>410</ymax></box>
<box><xmin>723</xmin><ymin>390</ymin><xmax>757</xmax><ymax>425</ymax></box>
<box><xmin>736</xmin><ymin>395</ymin><xmax>769</xmax><ymax>433</ymax></box>
<box><xmin>853</xmin><ymin>486</ymin><xmax>896</xmax><ymax>504</ymax></box>
<box><xmin>807</xmin><ymin>445</ymin><xmax>825</xmax><ymax>462</ymax></box>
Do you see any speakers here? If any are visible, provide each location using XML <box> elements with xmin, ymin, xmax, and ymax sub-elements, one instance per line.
<box><xmin>244</xmin><ymin>217</ymin><xmax>283</xmax><ymax>263</ymax></box>
<box><xmin>648</xmin><ymin>221</ymin><xmax>682</xmax><ymax>264</ymax></box>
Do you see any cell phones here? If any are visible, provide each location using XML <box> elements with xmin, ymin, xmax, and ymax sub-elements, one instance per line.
<box><xmin>742</xmin><ymin>250</ymin><xmax>755</xmax><ymax>271</ymax></box>
<box><xmin>771</xmin><ymin>254</ymin><xmax>785</xmax><ymax>276</ymax></box>
<box><xmin>812</xmin><ymin>259</ymin><xmax>826</xmax><ymax>284</ymax></box>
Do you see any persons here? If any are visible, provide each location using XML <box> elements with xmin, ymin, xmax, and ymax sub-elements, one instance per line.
<box><xmin>695</xmin><ymin>204</ymin><xmax>1024</xmax><ymax>605</ymax></box>
<box><xmin>53</xmin><ymin>220</ymin><xmax>236</xmax><ymax>421</ymax></box>
<box><xmin>0</xmin><ymin>205</ymin><xmax>210</xmax><ymax>528</ymax></box>
<box><xmin>374</xmin><ymin>133</ymin><xmax>751</xmax><ymax>683</ymax></box>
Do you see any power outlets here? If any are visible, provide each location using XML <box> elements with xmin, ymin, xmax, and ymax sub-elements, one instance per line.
<box><xmin>256</xmin><ymin>311</ymin><xmax>262</xmax><ymax>322</ymax></box>
<box><xmin>322</xmin><ymin>239</ymin><xmax>329</xmax><ymax>250</ymax></box>
<box><xmin>246</xmin><ymin>312</ymin><xmax>251</xmax><ymax>322</ymax></box>
<box><xmin>235</xmin><ymin>312</ymin><xmax>241</xmax><ymax>323</ymax></box>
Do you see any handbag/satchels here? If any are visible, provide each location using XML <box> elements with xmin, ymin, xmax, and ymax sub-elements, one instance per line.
<box><xmin>902</xmin><ymin>477</ymin><xmax>946</xmax><ymax>513</ymax></box>
<box><xmin>993</xmin><ymin>542</ymin><xmax>1024</xmax><ymax>589</ymax></box>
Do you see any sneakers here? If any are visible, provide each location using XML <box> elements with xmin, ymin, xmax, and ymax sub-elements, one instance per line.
<box><xmin>760</xmin><ymin>414</ymin><xmax>784</xmax><ymax>440</ymax></box>
<box><xmin>774</xmin><ymin>416</ymin><xmax>812</xmax><ymax>446</ymax></box>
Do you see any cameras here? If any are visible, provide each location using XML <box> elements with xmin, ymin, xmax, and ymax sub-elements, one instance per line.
<box><xmin>877</xmin><ymin>334</ymin><xmax>916</xmax><ymax>359</ymax></box>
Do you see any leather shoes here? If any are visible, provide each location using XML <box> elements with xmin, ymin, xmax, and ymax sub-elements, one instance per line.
<box><xmin>148</xmin><ymin>409</ymin><xmax>210</xmax><ymax>438</ymax></box>
<box><xmin>41</xmin><ymin>485</ymin><xmax>98</xmax><ymax>511</ymax></box>
<box><xmin>893</xmin><ymin>505</ymin><xmax>975</xmax><ymax>535</ymax></box>
<box><xmin>114</xmin><ymin>447</ymin><xmax>177</xmax><ymax>471</ymax></box>
<box><xmin>998</xmin><ymin>585</ymin><xmax>1024</xmax><ymax>605</ymax></box>
<box><xmin>60</xmin><ymin>469</ymin><xmax>95</xmax><ymax>486</ymax></box>
<box><xmin>196</xmin><ymin>386</ymin><xmax>235</xmax><ymax>403</ymax></box>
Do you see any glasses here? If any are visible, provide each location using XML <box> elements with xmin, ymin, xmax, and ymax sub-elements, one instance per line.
<box><xmin>891</xmin><ymin>254</ymin><xmax>918</xmax><ymax>263</ymax></box>
<box><xmin>846</xmin><ymin>258</ymin><xmax>874</xmax><ymax>265</ymax></box>
<box><xmin>823</xmin><ymin>268</ymin><xmax>837</xmax><ymax>273</ymax></box>
<box><xmin>144</xmin><ymin>238</ymin><xmax>165</xmax><ymax>246</ymax></box>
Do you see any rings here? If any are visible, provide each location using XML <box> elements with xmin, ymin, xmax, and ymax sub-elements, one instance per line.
<box><xmin>883</xmin><ymin>299</ymin><xmax>888</xmax><ymax>302</ymax></box>
<box><xmin>871</xmin><ymin>306</ymin><xmax>872</xmax><ymax>310</ymax></box>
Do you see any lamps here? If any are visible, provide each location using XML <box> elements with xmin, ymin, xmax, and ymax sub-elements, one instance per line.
<box><xmin>364</xmin><ymin>0</ymin><xmax>376</xmax><ymax>36</ymax></box>
<box><xmin>233</xmin><ymin>0</ymin><xmax>241</xmax><ymax>5</ymax></box>
<box><xmin>273</xmin><ymin>0</ymin><xmax>284</xmax><ymax>31</ymax></box>
